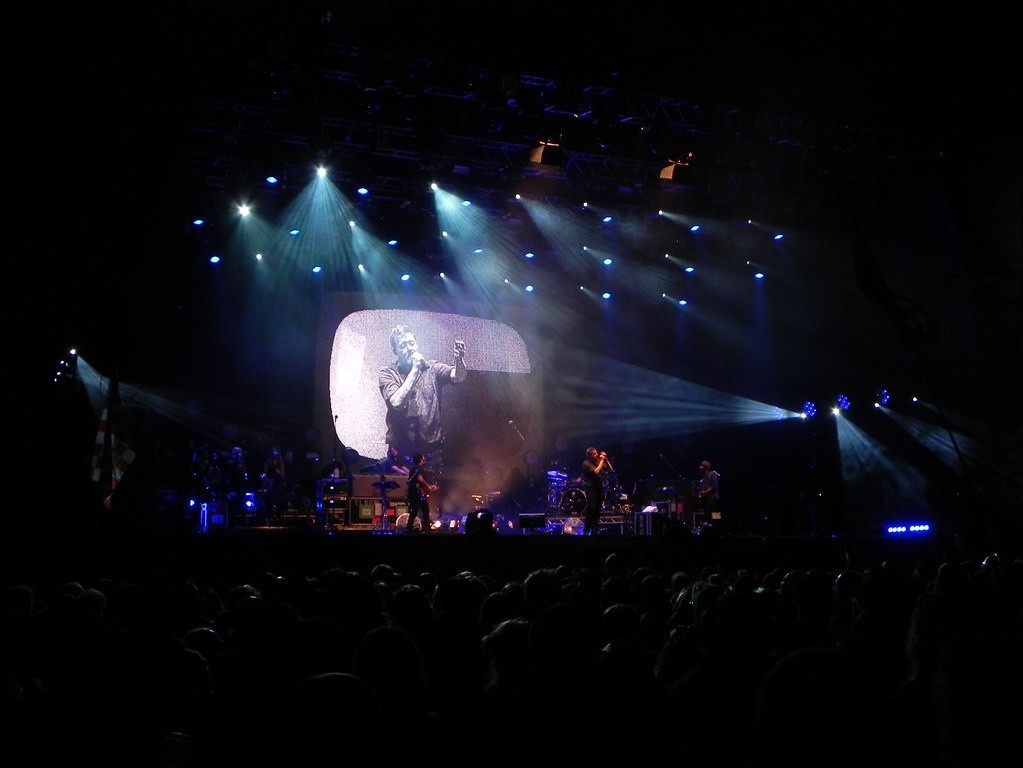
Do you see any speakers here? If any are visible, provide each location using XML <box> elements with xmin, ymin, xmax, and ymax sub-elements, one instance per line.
<box><xmin>384</xmin><ymin>475</ymin><xmax>410</xmax><ymax>499</ymax></box>
<box><xmin>351</xmin><ymin>474</ymin><xmax>382</xmax><ymax>497</ymax></box>
<box><xmin>519</xmin><ymin>513</ymin><xmax>546</xmax><ymax>528</ymax></box>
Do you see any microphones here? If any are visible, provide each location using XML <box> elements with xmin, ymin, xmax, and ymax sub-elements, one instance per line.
<box><xmin>420</xmin><ymin>359</ymin><xmax>430</xmax><ymax>371</ymax></box>
<box><xmin>599</xmin><ymin>456</ymin><xmax>609</xmax><ymax>460</ymax></box>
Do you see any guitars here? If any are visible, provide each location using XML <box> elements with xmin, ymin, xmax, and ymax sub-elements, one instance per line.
<box><xmin>419</xmin><ymin>484</ymin><xmax>436</xmax><ymax>501</ymax></box>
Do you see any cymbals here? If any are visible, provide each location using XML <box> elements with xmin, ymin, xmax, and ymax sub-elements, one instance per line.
<box><xmin>547</xmin><ymin>470</ymin><xmax>568</xmax><ymax>478</ymax></box>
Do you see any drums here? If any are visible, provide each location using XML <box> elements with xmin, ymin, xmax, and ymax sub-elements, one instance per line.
<box><xmin>562</xmin><ymin>516</ymin><xmax>592</xmax><ymax>536</ymax></box>
<box><xmin>559</xmin><ymin>488</ymin><xmax>588</xmax><ymax>516</ymax></box>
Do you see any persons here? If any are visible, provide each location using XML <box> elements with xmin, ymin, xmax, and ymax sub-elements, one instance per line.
<box><xmin>407</xmin><ymin>453</ymin><xmax>438</xmax><ymax>530</ymax></box>
<box><xmin>384</xmin><ymin>445</ymin><xmax>410</xmax><ymax>476</ymax></box>
<box><xmin>582</xmin><ymin>447</ymin><xmax>607</xmax><ymax>535</ymax></box>
<box><xmin>700</xmin><ymin>460</ymin><xmax>719</xmax><ymax>523</ymax></box>
<box><xmin>0</xmin><ymin>552</ymin><xmax>1023</xmax><ymax>732</ymax></box>
<box><xmin>378</xmin><ymin>324</ymin><xmax>468</xmax><ymax>461</ymax></box>
